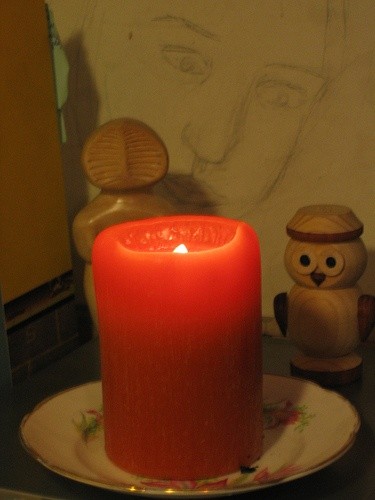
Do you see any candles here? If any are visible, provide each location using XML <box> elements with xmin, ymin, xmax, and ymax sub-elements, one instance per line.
<box><xmin>93</xmin><ymin>215</ymin><xmax>265</xmax><ymax>482</ymax></box>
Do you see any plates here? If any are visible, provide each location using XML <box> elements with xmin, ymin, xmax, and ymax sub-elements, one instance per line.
<box><xmin>19</xmin><ymin>372</ymin><xmax>361</xmax><ymax>499</ymax></box>
